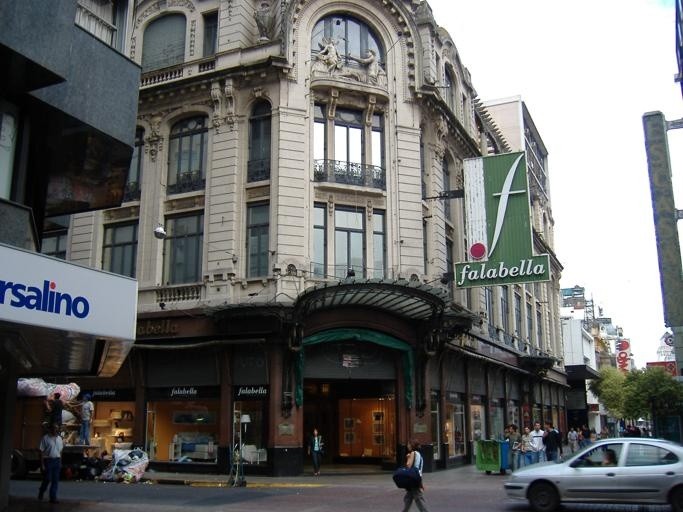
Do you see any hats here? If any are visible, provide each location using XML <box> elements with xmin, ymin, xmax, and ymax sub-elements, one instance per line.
<box><xmin>82</xmin><ymin>393</ymin><xmax>92</xmax><ymax>399</ymax></box>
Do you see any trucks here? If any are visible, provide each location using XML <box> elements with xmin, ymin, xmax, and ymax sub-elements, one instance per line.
<box><xmin>12</xmin><ymin>392</ymin><xmax>97</xmax><ymax>483</ymax></box>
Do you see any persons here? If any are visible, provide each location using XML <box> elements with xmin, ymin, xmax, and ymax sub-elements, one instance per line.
<box><xmin>400</xmin><ymin>438</ymin><xmax>430</xmax><ymax>511</ymax></box>
<box><xmin>67</xmin><ymin>393</ymin><xmax>94</xmax><ymax>445</ymax></box>
<box><xmin>45</xmin><ymin>392</ymin><xmax>63</xmax><ymax>433</ymax></box>
<box><xmin>584</xmin><ymin>448</ymin><xmax>616</xmax><ymax>467</ymax></box>
<box><xmin>36</xmin><ymin>424</ymin><xmax>65</xmax><ymax>504</ymax></box>
<box><xmin>306</xmin><ymin>427</ymin><xmax>324</xmax><ymax>475</ymax></box>
<box><xmin>567</xmin><ymin>423</ymin><xmax>610</xmax><ymax>454</ymax></box>
<box><xmin>622</xmin><ymin>424</ymin><xmax>649</xmax><ymax>457</ymax></box>
<box><xmin>505</xmin><ymin>420</ymin><xmax>563</xmax><ymax>478</ymax></box>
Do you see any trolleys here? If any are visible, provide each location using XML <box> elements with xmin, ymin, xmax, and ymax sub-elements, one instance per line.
<box><xmin>472</xmin><ymin>438</ymin><xmax>521</xmax><ymax>476</ymax></box>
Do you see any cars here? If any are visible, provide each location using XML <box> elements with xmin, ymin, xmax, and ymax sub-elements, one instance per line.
<box><xmin>505</xmin><ymin>436</ymin><xmax>682</xmax><ymax>512</ymax></box>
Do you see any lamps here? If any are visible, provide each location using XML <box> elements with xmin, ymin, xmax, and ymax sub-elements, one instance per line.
<box><xmin>151</xmin><ymin>222</ymin><xmax>167</xmax><ymax>239</ymax></box>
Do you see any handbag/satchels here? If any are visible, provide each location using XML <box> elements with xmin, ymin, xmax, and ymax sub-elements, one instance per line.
<box><xmin>393</xmin><ymin>467</ymin><xmax>421</xmax><ymax>488</ymax></box>
